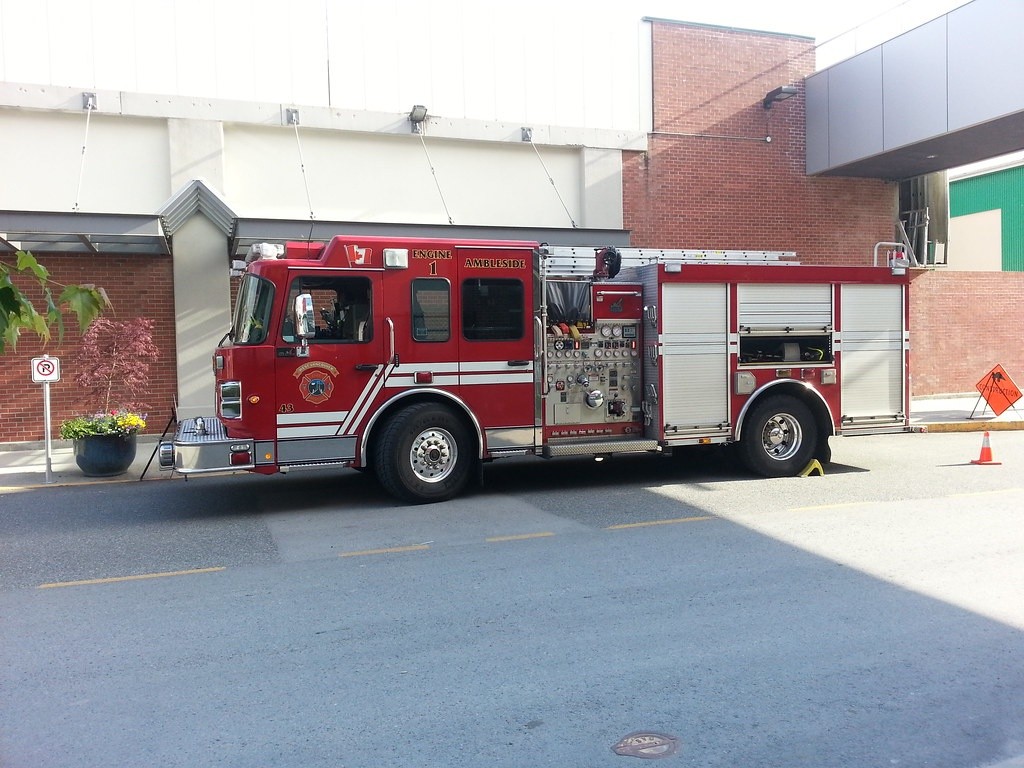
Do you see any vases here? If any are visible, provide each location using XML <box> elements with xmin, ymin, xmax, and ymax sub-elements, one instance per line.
<box><xmin>73</xmin><ymin>432</ymin><xmax>137</xmax><ymax>476</ymax></box>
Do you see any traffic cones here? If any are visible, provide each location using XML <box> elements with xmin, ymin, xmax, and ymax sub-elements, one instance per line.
<box><xmin>970</xmin><ymin>430</ymin><xmax>1002</xmax><ymax>465</ymax></box>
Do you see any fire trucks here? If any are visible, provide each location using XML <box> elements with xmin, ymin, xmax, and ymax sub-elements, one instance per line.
<box><xmin>159</xmin><ymin>234</ymin><xmax>911</xmax><ymax>504</ymax></box>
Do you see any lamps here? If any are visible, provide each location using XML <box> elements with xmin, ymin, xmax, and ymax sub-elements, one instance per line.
<box><xmin>764</xmin><ymin>86</ymin><xmax>798</xmax><ymax>109</ymax></box>
<box><xmin>408</xmin><ymin>105</ymin><xmax>428</xmax><ymax>123</ymax></box>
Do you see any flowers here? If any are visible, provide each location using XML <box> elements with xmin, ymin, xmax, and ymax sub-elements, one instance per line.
<box><xmin>58</xmin><ymin>408</ymin><xmax>146</xmax><ymax>440</ymax></box>
